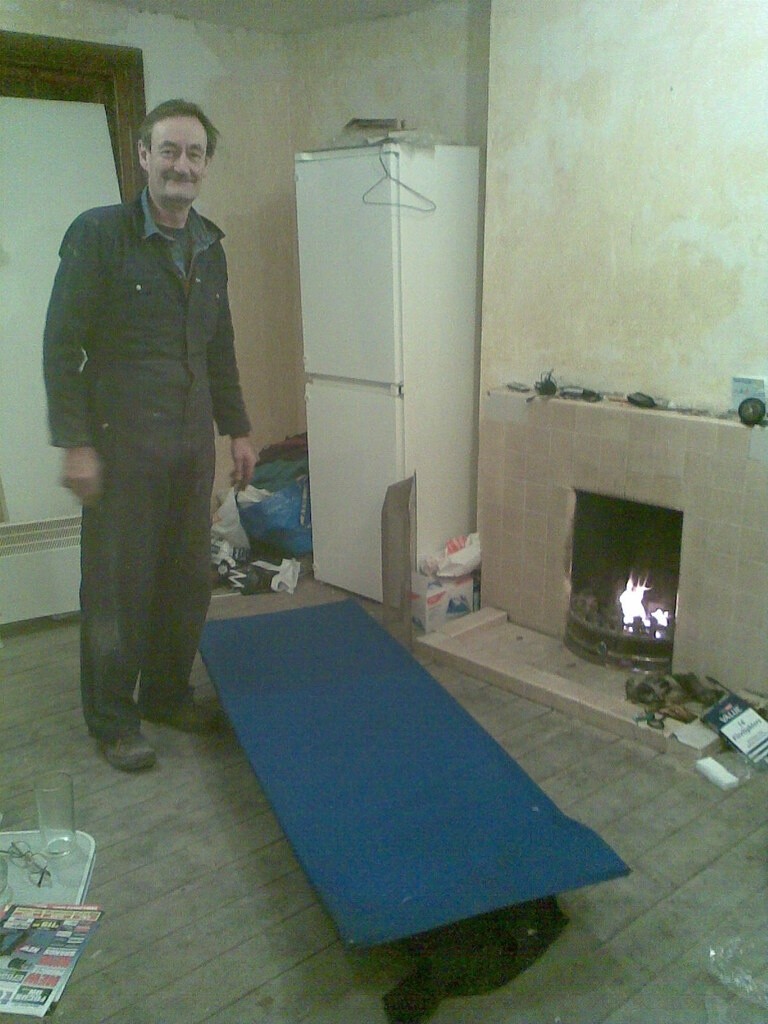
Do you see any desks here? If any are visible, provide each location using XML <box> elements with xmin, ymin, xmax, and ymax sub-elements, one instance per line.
<box><xmin>193</xmin><ymin>596</ymin><xmax>632</xmax><ymax>951</ymax></box>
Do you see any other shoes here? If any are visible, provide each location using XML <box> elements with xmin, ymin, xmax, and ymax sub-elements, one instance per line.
<box><xmin>98</xmin><ymin>729</ymin><xmax>157</xmax><ymax>769</ymax></box>
<box><xmin>138</xmin><ymin>700</ymin><xmax>221</xmax><ymax>734</ymax></box>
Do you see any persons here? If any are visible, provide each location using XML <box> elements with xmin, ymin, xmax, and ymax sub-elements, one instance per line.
<box><xmin>41</xmin><ymin>98</ymin><xmax>259</xmax><ymax>771</ymax></box>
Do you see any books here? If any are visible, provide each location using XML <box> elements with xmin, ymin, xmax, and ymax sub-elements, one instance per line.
<box><xmin>699</xmin><ymin>695</ymin><xmax>768</xmax><ymax>765</ymax></box>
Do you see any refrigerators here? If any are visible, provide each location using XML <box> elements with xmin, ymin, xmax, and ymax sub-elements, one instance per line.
<box><xmin>295</xmin><ymin>142</ymin><xmax>486</xmax><ymax>604</ymax></box>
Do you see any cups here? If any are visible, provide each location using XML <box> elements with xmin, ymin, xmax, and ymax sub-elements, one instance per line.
<box><xmin>32</xmin><ymin>771</ymin><xmax>78</xmax><ymax>857</ymax></box>
